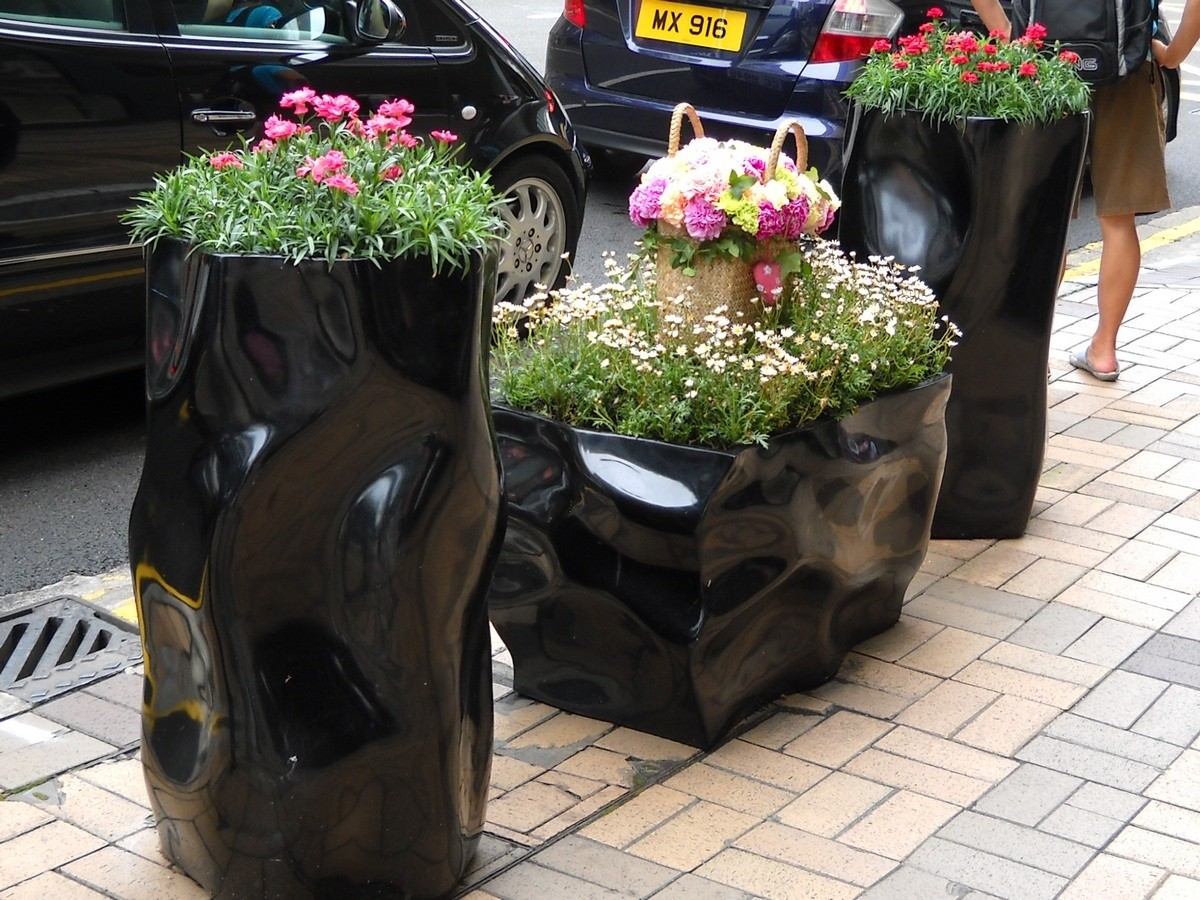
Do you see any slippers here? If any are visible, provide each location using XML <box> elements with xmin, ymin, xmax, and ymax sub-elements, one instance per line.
<box><xmin>1069</xmin><ymin>342</ymin><xmax>1122</xmax><ymax>380</ymax></box>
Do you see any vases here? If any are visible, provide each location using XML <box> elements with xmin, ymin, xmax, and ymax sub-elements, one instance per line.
<box><xmin>837</xmin><ymin>98</ymin><xmax>1090</xmax><ymax>540</ymax></box>
<box><xmin>130</xmin><ymin>232</ymin><xmax>510</xmax><ymax>899</ymax></box>
<box><xmin>492</xmin><ymin>372</ymin><xmax>953</xmax><ymax>753</ymax></box>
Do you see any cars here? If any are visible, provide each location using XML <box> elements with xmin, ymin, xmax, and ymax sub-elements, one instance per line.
<box><xmin>0</xmin><ymin>0</ymin><xmax>595</xmax><ymax>423</ymax></box>
<box><xmin>542</xmin><ymin>0</ymin><xmax>1181</xmax><ymax>247</ymax></box>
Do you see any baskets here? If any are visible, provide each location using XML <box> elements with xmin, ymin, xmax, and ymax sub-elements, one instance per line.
<box><xmin>655</xmin><ymin>104</ymin><xmax>808</xmax><ymax>334</ymax></box>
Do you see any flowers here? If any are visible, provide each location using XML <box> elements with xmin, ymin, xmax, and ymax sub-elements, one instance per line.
<box><xmin>838</xmin><ymin>7</ymin><xmax>1097</xmax><ymax>138</ymax></box>
<box><xmin>118</xmin><ymin>87</ymin><xmax>521</xmax><ymax>283</ymax></box>
<box><xmin>485</xmin><ymin>139</ymin><xmax>965</xmax><ymax>456</ymax></box>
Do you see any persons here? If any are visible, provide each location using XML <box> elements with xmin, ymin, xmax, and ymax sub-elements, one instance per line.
<box><xmin>972</xmin><ymin>0</ymin><xmax>1200</xmax><ymax>382</ymax></box>
<box><xmin>171</xmin><ymin>0</ymin><xmax>283</xmax><ymax>29</ymax></box>
